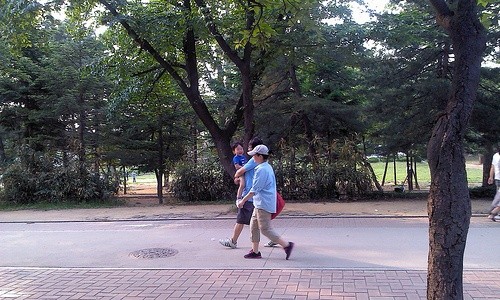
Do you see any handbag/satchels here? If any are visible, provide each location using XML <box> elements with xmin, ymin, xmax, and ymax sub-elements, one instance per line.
<box><xmin>271</xmin><ymin>193</ymin><xmax>285</xmax><ymax>220</ymax></box>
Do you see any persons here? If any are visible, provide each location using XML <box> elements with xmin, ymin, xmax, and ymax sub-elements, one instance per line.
<box><xmin>230</xmin><ymin>141</ymin><xmax>248</xmax><ymax>208</ymax></box>
<box><xmin>218</xmin><ymin>138</ymin><xmax>279</xmax><ymax>249</ymax></box>
<box><xmin>132</xmin><ymin>170</ymin><xmax>136</xmax><ymax>182</ymax></box>
<box><xmin>487</xmin><ymin>146</ymin><xmax>500</xmax><ymax>223</ymax></box>
<box><xmin>244</xmin><ymin>145</ymin><xmax>295</xmax><ymax>260</ymax></box>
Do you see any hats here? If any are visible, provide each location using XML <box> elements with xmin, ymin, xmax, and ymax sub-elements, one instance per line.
<box><xmin>247</xmin><ymin>144</ymin><xmax>269</xmax><ymax>156</ymax></box>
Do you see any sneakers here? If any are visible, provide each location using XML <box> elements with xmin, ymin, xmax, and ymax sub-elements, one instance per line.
<box><xmin>264</xmin><ymin>240</ymin><xmax>277</xmax><ymax>247</ymax></box>
<box><xmin>244</xmin><ymin>251</ymin><xmax>261</xmax><ymax>258</ymax></box>
<box><xmin>219</xmin><ymin>238</ymin><xmax>237</xmax><ymax>249</ymax></box>
<box><xmin>283</xmin><ymin>241</ymin><xmax>294</xmax><ymax>260</ymax></box>
